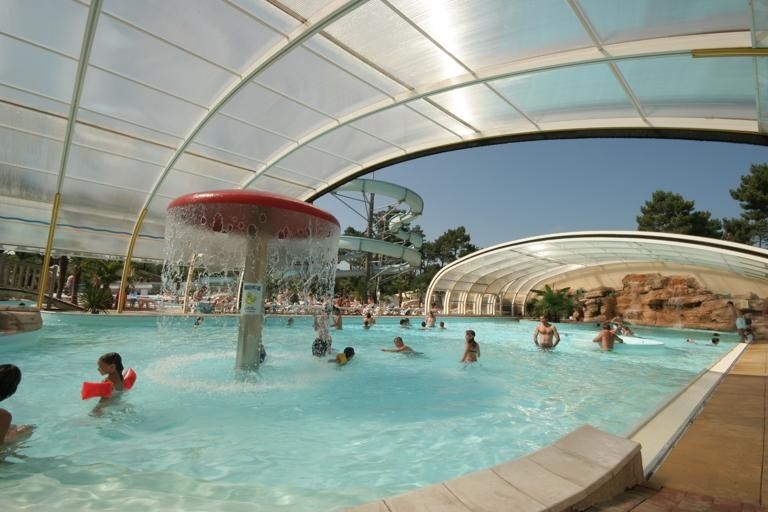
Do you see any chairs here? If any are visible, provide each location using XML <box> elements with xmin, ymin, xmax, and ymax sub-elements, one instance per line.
<box><xmin>180</xmin><ymin>292</ymin><xmax>439</xmax><ymax>317</ymax></box>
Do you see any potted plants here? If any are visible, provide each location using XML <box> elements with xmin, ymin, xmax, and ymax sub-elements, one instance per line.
<box><xmin>530</xmin><ymin>281</ymin><xmax>582</xmax><ymax>322</ymax></box>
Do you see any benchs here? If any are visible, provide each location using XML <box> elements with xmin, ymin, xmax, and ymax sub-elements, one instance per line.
<box><xmin>112</xmin><ymin>295</ymin><xmax>161</xmax><ymax>310</ymax></box>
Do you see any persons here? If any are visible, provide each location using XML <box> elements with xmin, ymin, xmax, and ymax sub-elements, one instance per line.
<box><xmin>259</xmin><ymin>282</ymin><xmax>447</xmax><ymax>365</ymax></box>
<box><xmin>0</xmin><ymin>364</ymin><xmax>33</xmax><ymax>441</ymax></box>
<box><xmin>460</xmin><ymin>330</ymin><xmax>480</xmax><ymax>361</ymax></box>
<box><xmin>22</xmin><ymin>262</ymin><xmax>75</xmax><ymax>296</ymax></box>
<box><xmin>533</xmin><ymin>314</ymin><xmax>560</xmax><ymax>347</ymax></box>
<box><xmin>115</xmin><ymin>277</ymin><xmax>237</xmax><ymax>329</ymax></box>
<box><xmin>572</xmin><ymin>308</ymin><xmax>633</xmax><ymax>350</ymax></box>
<box><xmin>687</xmin><ymin>301</ymin><xmax>755</xmax><ymax>345</ymax></box>
<box><xmin>82</xmin><ymin>352</ymin><xmax>136</xmax><ymax>417</ymax></box>
